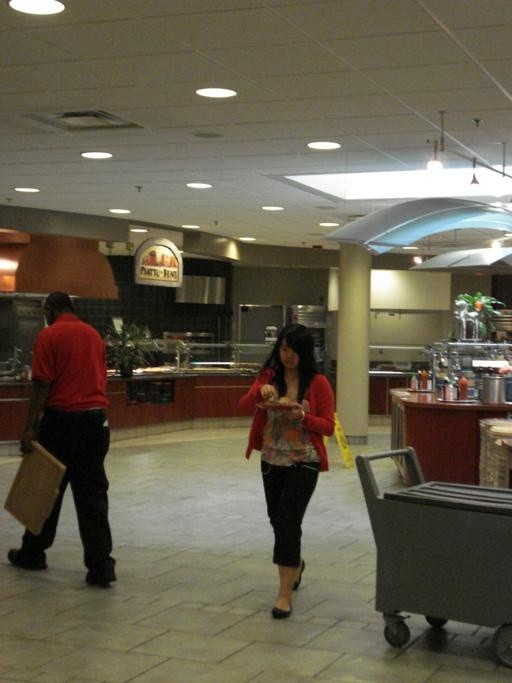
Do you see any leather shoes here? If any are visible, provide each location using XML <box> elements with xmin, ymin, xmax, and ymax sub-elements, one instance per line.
<box><xmin>7</xmin><ymin>549</ymin><xmax>47</xmax><ymax>570</ymax></box>
<box><xmin>85</xmin><ymin>569</ymin><xmax>116</xmax><ymax>585</ymax></box>
<box><xmin>272</xmin><ymin>607</ymin><xmax>292</xmax><ymax>619</ymax></box>
<box><xmin>293</xmin><ymin>560</ymin><xmax>305</xmax><ymax>590</ymax></box>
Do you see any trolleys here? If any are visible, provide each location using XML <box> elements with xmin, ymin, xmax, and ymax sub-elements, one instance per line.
<box><xmin>354</xmin><ymin>442</ymin><xmax>512</xmax><ymax>666</ymax></box>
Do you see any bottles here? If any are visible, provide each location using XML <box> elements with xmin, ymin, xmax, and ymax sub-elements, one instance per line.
<box><xmin>412</xmin><ymin>368</ymin><xmax>477</xmax><ymax>401</ymax></box>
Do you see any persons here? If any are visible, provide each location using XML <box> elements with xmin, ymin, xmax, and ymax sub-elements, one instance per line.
<box><xmin>7</xmin><ymin>289</ymin><xmax>120</xmax><ymax>587</ymax></box>
<box><xmin>236</xmin><ymin>321</ymin><xmax>338</xmax><ymax>618</ymax></box>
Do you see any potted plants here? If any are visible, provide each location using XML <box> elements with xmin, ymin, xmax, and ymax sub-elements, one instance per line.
<box><xmin>105</xmin><ymin>322</ymin><xmax>161</xmax><ymax>378</ymax></box>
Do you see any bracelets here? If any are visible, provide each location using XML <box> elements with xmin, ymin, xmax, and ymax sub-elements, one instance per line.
<box><xmin>295</xmin><ymin>410</ymin><xmax>306</xmax><ymax>422</ymax></box>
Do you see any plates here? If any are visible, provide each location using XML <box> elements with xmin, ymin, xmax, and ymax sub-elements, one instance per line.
<box><xmin>255</xmin><ymin>401</ymin><xmax>301</xmax><ymax>412</ymax></box>
<box><xmin>492</xmin><ymin>309</ymin><xmax>512</xmax><ymax>332</ymax></box>
